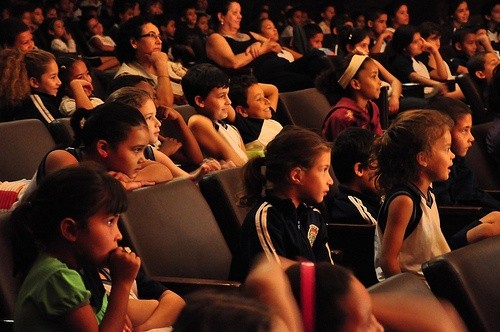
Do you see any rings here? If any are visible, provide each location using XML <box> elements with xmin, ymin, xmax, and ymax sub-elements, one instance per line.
<box><xmin>123</xmin><ymin>247</ymin><xmax>132</xmax><ymax>254</ymax></box>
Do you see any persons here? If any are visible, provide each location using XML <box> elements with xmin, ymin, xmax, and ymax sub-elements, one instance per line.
<box><xmin>0</xmin><ymin>0</ymin><xmax>500</xmax><ymax>332</ymax></box>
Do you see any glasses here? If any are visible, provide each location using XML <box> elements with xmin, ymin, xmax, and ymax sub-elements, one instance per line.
<box><xmin>140</xmin><ymin>33</ymin><xmax>162</xmax><ymax>40</ymax></box>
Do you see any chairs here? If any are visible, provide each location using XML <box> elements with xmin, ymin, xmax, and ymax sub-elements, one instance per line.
<box><xmin>0</xmin><ymin>84</ymin><xmax>500</xmax><ymax>332</ymax></box>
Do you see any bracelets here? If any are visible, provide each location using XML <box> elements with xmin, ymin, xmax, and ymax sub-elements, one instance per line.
<box><xmin>157</xmin><ymin>73</ymin><xmax>170</xmax><ymax>79</ymax></box>
